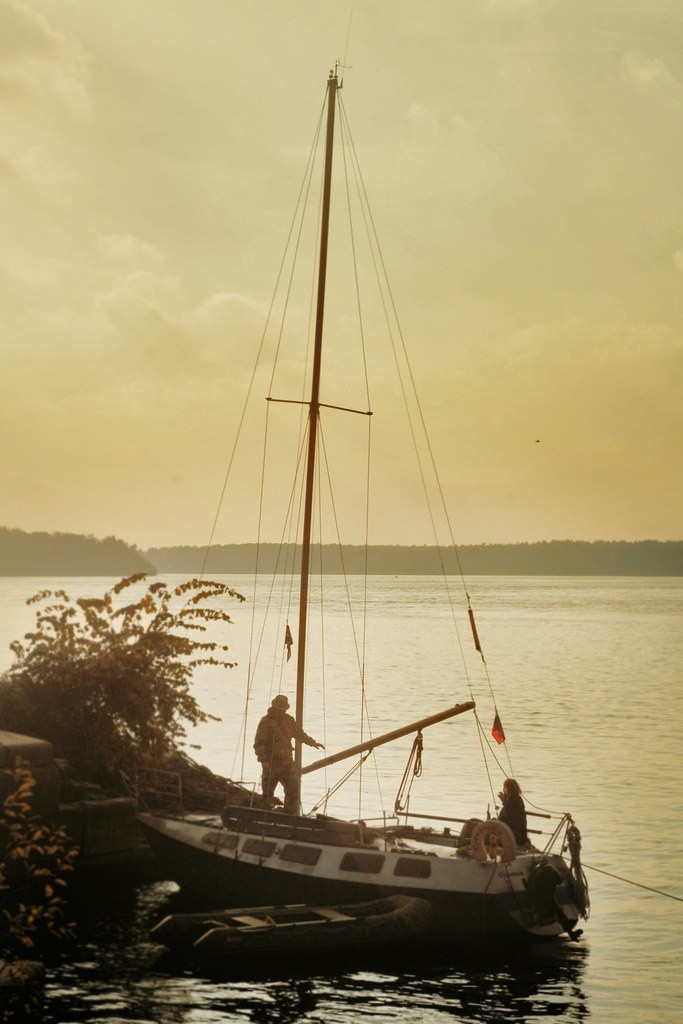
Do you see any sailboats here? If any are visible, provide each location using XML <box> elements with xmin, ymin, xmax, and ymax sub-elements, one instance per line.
<box><xmin>139</xmin><ymin>66</ymin><xmax>583</xmax><ymax>943</ymax></box>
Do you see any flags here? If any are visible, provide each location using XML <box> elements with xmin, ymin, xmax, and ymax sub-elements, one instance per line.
<box><xmin>286</xmin><ymin>627</ymin><xmax>293</xmax><ymax>661</ymax></box>
<box><xmin>492</xmin><ymin>718</ymin><xmax>506</xmax><ymax>744</ymax></box>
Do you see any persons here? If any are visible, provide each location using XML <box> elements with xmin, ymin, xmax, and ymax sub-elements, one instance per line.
<box><xmin>252</xmin><ymin>695</ymin><xmax>324</xmax><ymax>814</ymax></box>
<box><xmin>497</xmin><ymin>779</ymin><xmax>527</xmax><ymax>845</ymax></box>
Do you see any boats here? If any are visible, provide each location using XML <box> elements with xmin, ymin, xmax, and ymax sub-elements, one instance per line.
<box><xmin>145</xmin><ymin>895</ymin><xmax>434</xmax><ymax>970</ymax></box>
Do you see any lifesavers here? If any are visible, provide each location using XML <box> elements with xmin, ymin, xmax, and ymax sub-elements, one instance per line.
<box><xmin>468</xmin><ymin>820</ymin><xmax>517</xmax><ymax>869</ymax></box>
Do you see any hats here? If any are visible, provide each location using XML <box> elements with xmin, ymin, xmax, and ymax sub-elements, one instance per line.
<box><xmin>271</xmin><ymin>695</ymin><xmax>290</xmax><ymax>710</ymax></box>
